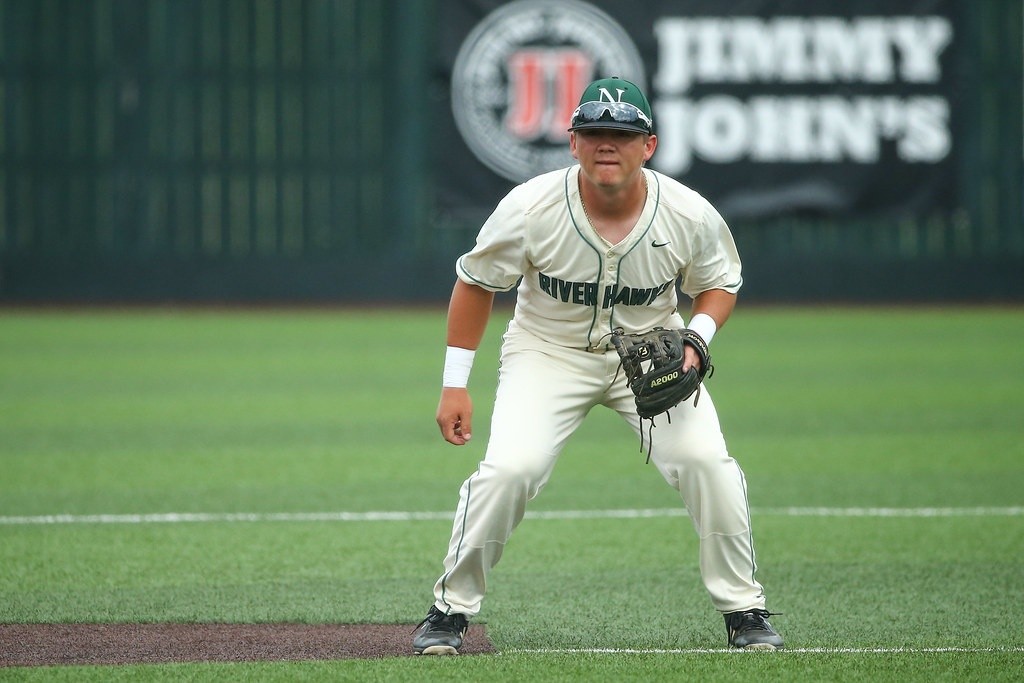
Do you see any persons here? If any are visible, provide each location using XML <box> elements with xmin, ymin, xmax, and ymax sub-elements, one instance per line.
<box><xmin>411</xmin><ymin>77</ymin><xmax>785</xmax><ymax>653</ymax></box>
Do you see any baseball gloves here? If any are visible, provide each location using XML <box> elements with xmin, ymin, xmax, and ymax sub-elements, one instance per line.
<box><xmin>592</xmin><ymin>326</ymin><xmax>715</xmax><ymax>465</ymax></box>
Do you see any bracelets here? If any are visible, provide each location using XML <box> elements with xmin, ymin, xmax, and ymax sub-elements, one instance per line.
<box><xmin>687</xmin><ymin>314</ymin><xmax>717</xmax><ymax>346</ymax></box>
<box><xmin>443</xmin><ymin>346</ymin><xmax>476</xmax><ymax>388</ymax></box>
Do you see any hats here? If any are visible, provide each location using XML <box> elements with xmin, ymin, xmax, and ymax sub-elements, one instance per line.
<box><xmin>567</xmin><ymin>77</ymin><xmax>652</xmax><ymax>135</ymax></box>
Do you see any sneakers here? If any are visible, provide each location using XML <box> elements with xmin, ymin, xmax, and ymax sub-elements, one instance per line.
<box><xmin>408</xmin><ymin>605</ymin><xmax>469</xmax><ymax>655</ymax></box>
<box><xmin>723</xmin><ymin>610</ymin><xmax>784</xmax><ymax>650</ymax></box>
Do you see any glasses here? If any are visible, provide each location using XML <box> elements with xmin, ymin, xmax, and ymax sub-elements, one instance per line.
<box><xmin>570</xmin><ymin>99</ymin><xmax>651</xmax><ymax>132</ymax></box>
